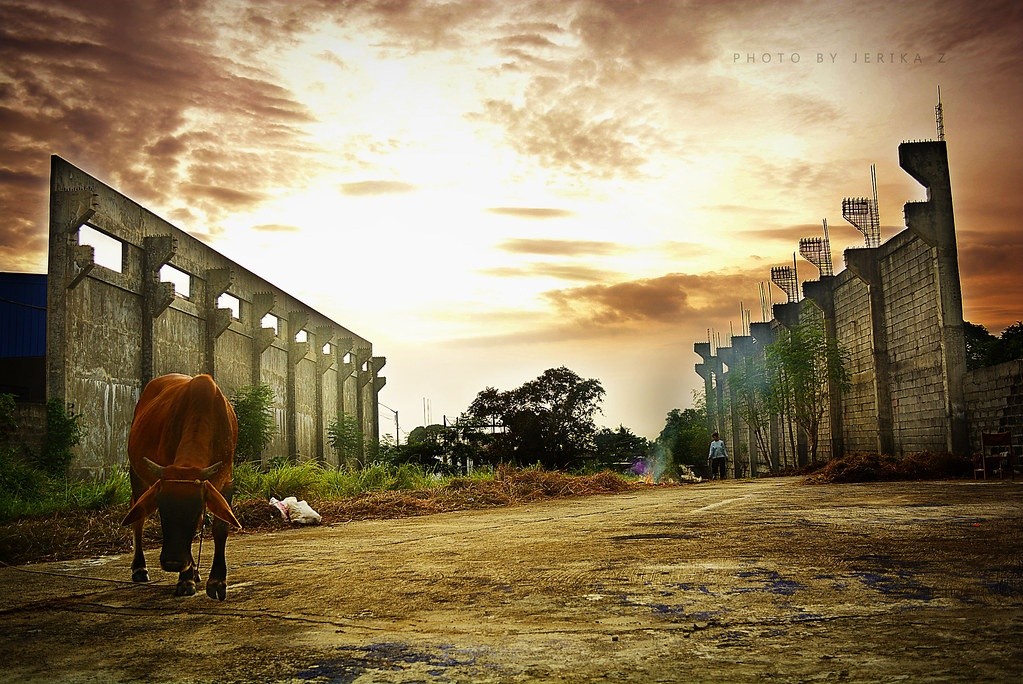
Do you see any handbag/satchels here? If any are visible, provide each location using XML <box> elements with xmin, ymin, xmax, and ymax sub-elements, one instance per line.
<box><xmin>269</xmin><ymin>496</ymin><xmax>321</xmax><ymax>523</ymax></box>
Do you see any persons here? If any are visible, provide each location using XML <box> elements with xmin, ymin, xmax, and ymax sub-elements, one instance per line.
<box><xmin>707</xmin><ymin>432</ymin><xmax>729</xmax><ymax>480</ymax></box>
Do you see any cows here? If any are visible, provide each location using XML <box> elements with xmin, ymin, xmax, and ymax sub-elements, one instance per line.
<box><xmin>120</xmin><ymin>371</ymin><xmax>244</xmax><ymax>600</ymax></box>
